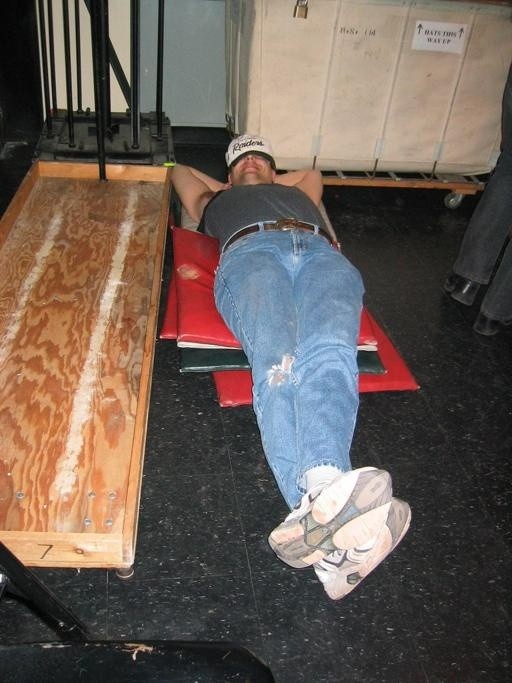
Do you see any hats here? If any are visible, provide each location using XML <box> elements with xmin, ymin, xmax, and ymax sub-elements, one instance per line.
<box><xmin>225</xmin><ymin>134</ymin><xmax>276</xmax><ymax>170</ymax></box>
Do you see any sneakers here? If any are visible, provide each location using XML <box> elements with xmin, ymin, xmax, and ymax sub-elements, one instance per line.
<box><xmin>313</xmin><ymin>497</ymin><xmax>413</xmax><ymax>600</ymax></box>
<box><xmin>268</xmin><ymin>466</ymin><xmax>394</xmax><ymax>568</ymax></box>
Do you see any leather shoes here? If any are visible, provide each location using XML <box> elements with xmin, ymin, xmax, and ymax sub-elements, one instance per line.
<box><xmin>443</xmin><ymin>272</ymin><xmax>480</xmax><ymax>306</ymax></box>
<box><xmin>471</xmin><ymin>311</ymin><xmax>512</xmax><ymax>336</ymax></box>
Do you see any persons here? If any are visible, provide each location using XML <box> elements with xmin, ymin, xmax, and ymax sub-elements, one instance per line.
<box><xmin>168</xmin><ymin>131</ymin><xmax>415</xmax><ymax>603</ymax></box>
<box><xmin>440</xmin><ymin>62</ymin><xmax>512</xmax><ymax>338</ymax></box>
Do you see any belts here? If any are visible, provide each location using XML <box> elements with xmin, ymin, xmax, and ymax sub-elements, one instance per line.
<box><xmin>222</xmin><ymin>217</ymin><xmax>333</xmax><ymax>252</ymax></box>
